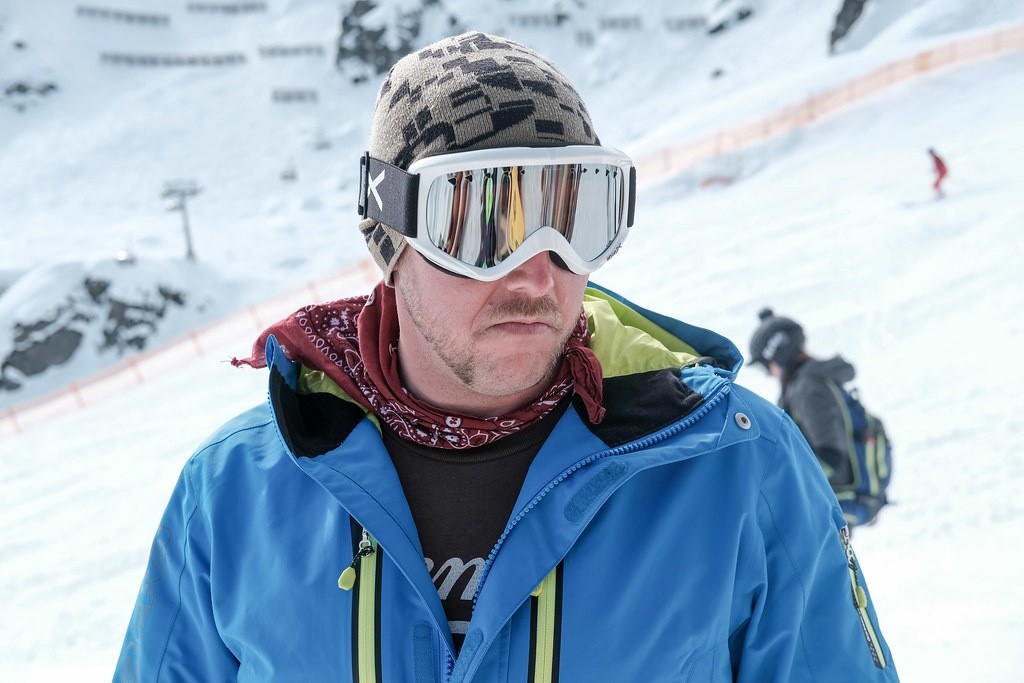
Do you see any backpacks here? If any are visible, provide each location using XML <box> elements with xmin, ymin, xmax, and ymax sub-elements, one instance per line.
<box><xmin>819</xmin><ymin>378</ymin><xmax>892</xmax><ymax>525</ymax></box>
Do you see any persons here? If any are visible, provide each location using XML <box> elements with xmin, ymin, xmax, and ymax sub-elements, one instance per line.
<box><xmin>928</xmin><ymin>149</ymin><xmax>949</xmax><ymax>200</ymax></box>
<box><xmin>111</xmin><ymin>32</ymin><xmax>900</xmax><ymax>683</ymax></box>
<box><xmin>744</xmin><ymin>307</ymin><xmax>892</xmax><ymax>539</ymax></box>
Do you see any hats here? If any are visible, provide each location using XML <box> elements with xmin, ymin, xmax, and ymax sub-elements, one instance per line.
<box><xmin>358</xmin><ymin>31</ymin><xmax>600</xmax><ymax>287</ymax></box>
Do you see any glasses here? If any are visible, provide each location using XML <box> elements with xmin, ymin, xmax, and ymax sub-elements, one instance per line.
<box><xmin>400</xmin><ymin>144</ymin><xmax>638</xmax><ymax>283</ymax></box>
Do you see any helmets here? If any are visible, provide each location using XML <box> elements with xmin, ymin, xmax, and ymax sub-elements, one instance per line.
<box><xmin>747</xmin><ymin>315</ymin><xmax>803</xmax><ymax>367</ymax></box>
